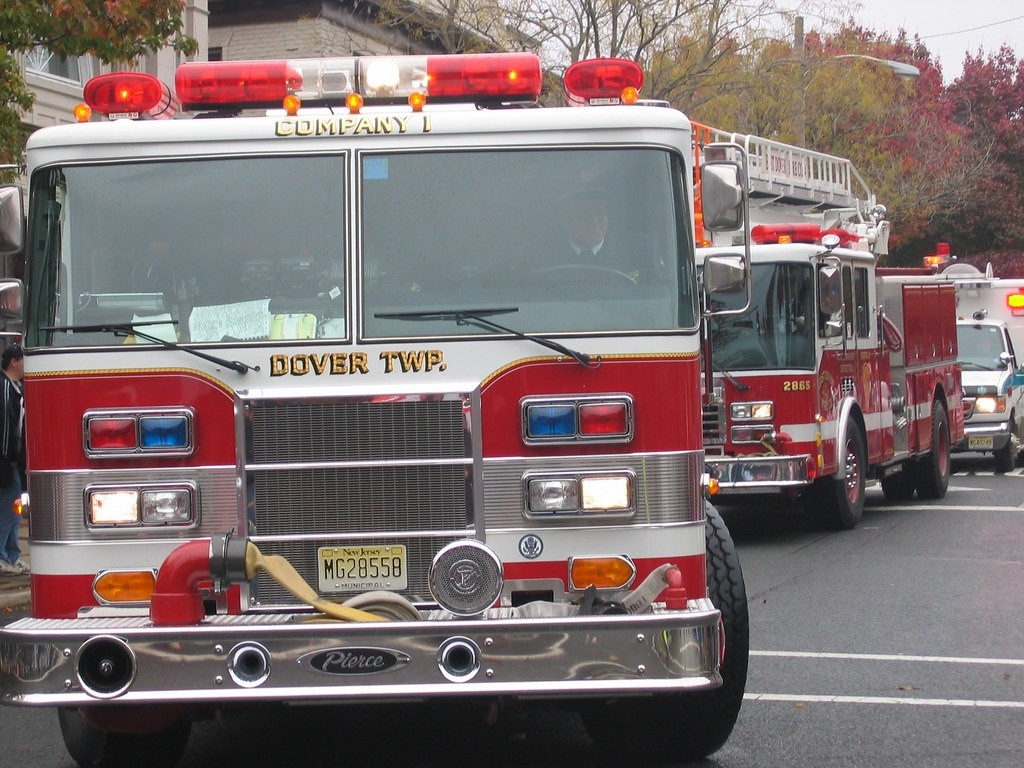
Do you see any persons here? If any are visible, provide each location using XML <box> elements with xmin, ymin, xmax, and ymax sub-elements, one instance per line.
<box><xmin>524</xmin><ymin>174</ymin><xmax>653</xmax><ymax>300</ymax></box>
<box><xmin>0</xmin><ymin>343</ymin><xmax>30</xmax><ymax>577</ymax></box>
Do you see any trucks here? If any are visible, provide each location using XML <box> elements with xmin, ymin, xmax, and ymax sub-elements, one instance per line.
<box><xmin>950</xmin><ymin>279</ymin><xmax>1024</xmax><ymax>473</ymax></box>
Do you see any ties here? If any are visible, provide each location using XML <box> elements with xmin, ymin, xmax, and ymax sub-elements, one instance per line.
<box><xmin>580</xmin><ymin>250</ymin><xmax>593</xmax><ymax>263</ymax></box>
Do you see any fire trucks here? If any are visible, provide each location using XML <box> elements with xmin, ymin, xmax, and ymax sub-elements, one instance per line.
<box><xmin>1</xmin><ymin>53</ymin><xmax>750</xmax><ymax>768</ymax></box>
<box><xmin>688</xmin><ymin>119</ymin><xmax>962</xmax><ymax>532</ymax></box>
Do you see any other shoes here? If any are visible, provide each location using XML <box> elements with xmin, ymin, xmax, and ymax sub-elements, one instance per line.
<box><xmin>13</xmin><ymin>561</ymin><xmax>30</xmax><ymax>575</ymax></box>
<box><xmin>0</xmin><ymin>560</ymin><xmax>18</xmax><ymax>576</ymax></box>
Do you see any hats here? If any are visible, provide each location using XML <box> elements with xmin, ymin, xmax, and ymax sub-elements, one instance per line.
<box><xmin>1</xmin><ymin>344</ymin><xmax>22</xmax><ymax>358</ymax></box>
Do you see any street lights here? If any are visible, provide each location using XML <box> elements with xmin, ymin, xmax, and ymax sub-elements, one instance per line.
<box><xmin>795</xmin><ymin>54</ymin><xmax>921</xmax><ymax>148</ymax></box>
